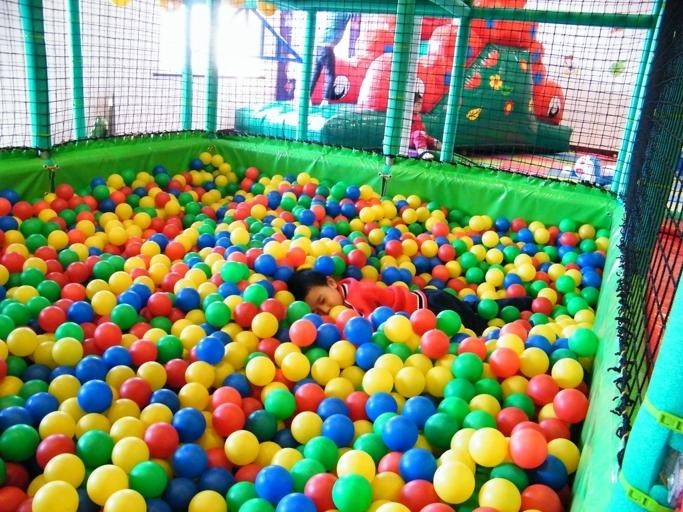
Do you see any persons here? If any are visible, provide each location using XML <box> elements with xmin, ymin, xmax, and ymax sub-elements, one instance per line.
<box><xmin>287</xmin><ymin>267</ymin><xmax>538</xmax><ymax>338</ymax></box>
<box><xmin>409</xmin><ymin>94</ymin><xmax>443</xmax><ymax>161</ymax></box>
<box><xmin>310</xmin><ymin>10</ymin><xmax>354</xmax><ymax>107</ymax></box>
